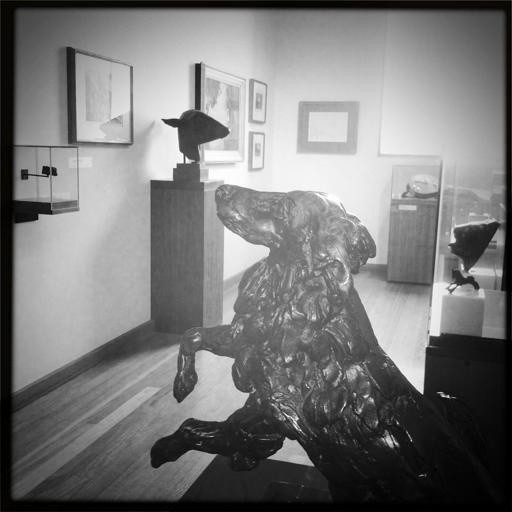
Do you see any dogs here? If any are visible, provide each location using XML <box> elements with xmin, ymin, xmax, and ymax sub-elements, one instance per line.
<box><xmin>151</xmin><ymin>184</ymin><xmax>506</xmax><ymax>501</ymax></box>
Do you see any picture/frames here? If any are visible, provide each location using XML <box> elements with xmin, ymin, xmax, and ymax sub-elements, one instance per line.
<box><xmin>66</xmin><ymin>43</ymin><xmax>135</xmax><ymax>146</ymax></box>
<box><xmin>296</xmin><ymin>100</ymin><xmax>361</xmax><ymax>155</ymax></box>
<box><xmin>247</xmin><ymin>131</ymin><xmax>265</xmax><ymax>170</ymax></box>
<box><xmin>248</xmin><ymin>77</ymin><xmax>268</xmax><ymax>123</ymax></box>
<box><xmin>197</xmin><ymin>60</ymin><xmax>246</xmax><ymax>165</ymax></box>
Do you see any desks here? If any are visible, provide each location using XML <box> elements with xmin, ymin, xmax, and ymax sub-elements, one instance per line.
<box><xmin>423</xmin><ymin>282</ymin><xmax>509</xmax><ymax>437</ymax></box>
